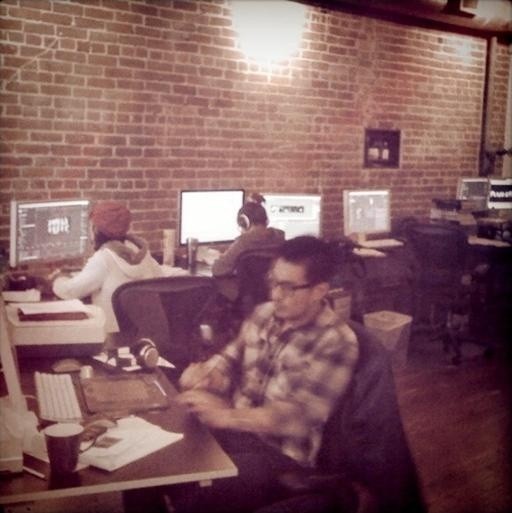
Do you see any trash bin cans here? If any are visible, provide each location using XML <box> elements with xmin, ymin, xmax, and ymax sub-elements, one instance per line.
<box><xmin>364</xmin><ymin>311</ymin><xmax>413</xmax><ymax>369</ymax></box>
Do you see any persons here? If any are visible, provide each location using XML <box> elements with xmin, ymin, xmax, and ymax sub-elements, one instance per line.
<box><xmin>211</xmin><ymin>191</ymin><xmax>286</xmax><ymax>280</ymax></box>
<box><xmin>122</xmin><ymin>234</ymin><xmax>360</xmax><ymax>512</ymax></box>
<box><xmin>46</xmin><ymin>201</ymin><xmax>164</xmax><ymax>337</ymax></box>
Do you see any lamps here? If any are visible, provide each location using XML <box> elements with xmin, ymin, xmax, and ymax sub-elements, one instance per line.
<box><xmin>368</xmin><ymin>136</ymin><xmax>390</xmax><ymax>168</ymax></box>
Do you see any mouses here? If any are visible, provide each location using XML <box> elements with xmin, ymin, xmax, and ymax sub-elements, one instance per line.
<box><xmin>51</xmin><ymin>358</ymin><xmax>83</xmax><ymax>372</ymax></box>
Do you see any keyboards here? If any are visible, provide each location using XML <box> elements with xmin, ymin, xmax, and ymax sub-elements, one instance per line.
<box><xmin>358</xmin><ymin>238</ymin><xmax>405</xmax><ymax>248</ymax></box>
<box><xmin>34</xmin><ymin>371</ymin><xmax>85</xmax><ymax>425</ymax></box>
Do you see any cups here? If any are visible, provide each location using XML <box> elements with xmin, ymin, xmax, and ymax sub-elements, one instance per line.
<box><xmin>186</xmin><ymin>237</ymin><xmax>201</xmax><ymax>266</ymax></box>
<box><xmin>44</xmin><ymin>422</ymin><xmax>97</xmax><ymax>475</ymax></box>
<box><xmin>162</xmin><ymin>228</ymin><xmax>175</xmax><ymax>265</ymax></box>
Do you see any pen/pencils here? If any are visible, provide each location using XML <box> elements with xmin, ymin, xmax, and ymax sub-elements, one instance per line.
<box><xmin>154</xmin><ymin>379</ymin><xmax>168</xmax><ymax>398</ymax></box>
<box><xmin>22</xmin><ymin>466</ymin><xmax>47</xmax><ymax>479</ymax></box>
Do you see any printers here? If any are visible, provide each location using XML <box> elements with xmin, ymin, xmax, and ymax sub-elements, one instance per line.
<box><xmin>3</xmin><ymin>303</ymin><xmax>106</xmax><ymax>357</ymax></box>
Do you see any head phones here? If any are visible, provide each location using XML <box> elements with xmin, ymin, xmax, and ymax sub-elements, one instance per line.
<box><xmin>238</xmin><ymin>211</ymin><xmax>270</xmax><ymax>230</ymax></box>
<box><xmin>130</xmin><ymin>337</ymin><xmax>158</xmax><ymax>372</ymax></box>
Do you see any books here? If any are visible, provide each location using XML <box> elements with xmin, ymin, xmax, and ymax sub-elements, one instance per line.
<box><xmin>79</xmin><ymin>415</ymin><xmax>185</xmax><ymax>472</ymax></box>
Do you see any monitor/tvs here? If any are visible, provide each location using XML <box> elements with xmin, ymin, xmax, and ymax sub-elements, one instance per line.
<box><xmin>341</xmin><ymin>188</ymin><xmax>392</xmax><ymax>241</ymax></box>
<box><xmin>9</xmin><ymin>198</ymin><xmax>91</xmax><ymax>271</ymax></box>
<box><xmin>487</xmin><ymin>177</ymin><xmax>512</xmax><ymax>211</ymax></box>
<box><xmin>1</xmin><ymin>301</ymin><xmax>39</xmax><ymax>429</ymax></box>
<box><xmin>260</xmin><ymin>193</ymin><xmax>323</xmax><ymax>242</ymax></box>
<box><xmin>454</xmin><ymin>176</ymin><xmax>489</xmax><ymax>213</ymax></box>
<box><xmin>177</xmin><ymin>189</ymin><xmax>246</xmax><ymax>247</ymax></box>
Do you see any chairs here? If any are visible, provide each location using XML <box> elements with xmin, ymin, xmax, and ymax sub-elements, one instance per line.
<box><xmin>238</xmin><ymin>317</ymin><xmax>390</xmax><ymax>512</ymax></box>
<box><xmin>111</xmin><ymin>276</ymin><xmax>221</xmax><ymax>394</ymax></box>
<box><xmin>234</xmin><ymin>246</ymin><xmax>279</xmax><ymax>322</ymax></box>
<box><xmin>407</xmin><ymin>223</ymin><xmax>490</xmax><ymax>365</ymax></box>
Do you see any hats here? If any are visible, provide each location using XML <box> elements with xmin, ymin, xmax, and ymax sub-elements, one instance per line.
<box><xmin>88</xmin><ymin>201</ymin><xmax>133</xmax><ymax>239</ymax></box>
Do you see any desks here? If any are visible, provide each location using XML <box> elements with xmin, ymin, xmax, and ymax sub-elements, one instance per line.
<box><xmin>1</xmin><ymin>331</ymin><xmax>239</xmax><ymax>512</ymax></box>
<box><xmin>466</xmin><ymin>236</ymin><xmax>511</xmax><ymax>356</ymax></box>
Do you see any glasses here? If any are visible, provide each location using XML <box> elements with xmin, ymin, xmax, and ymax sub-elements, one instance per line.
<box><xmin>262</xmin><ymin>269</ymin><xmax>313</xmax><ymax>297</ymax></box>
